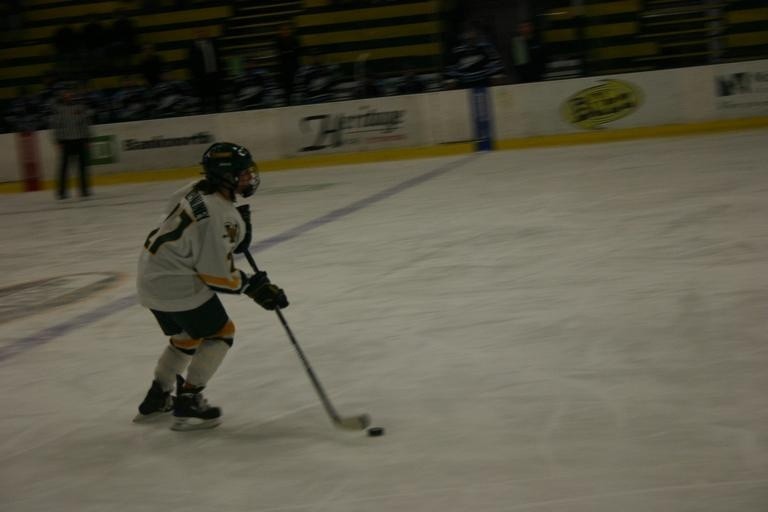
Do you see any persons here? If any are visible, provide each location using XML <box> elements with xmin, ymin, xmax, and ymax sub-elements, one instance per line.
<box><xmin>135</xmin><ymin>141</ymin><xmax>290</xmax><ymax>419</ymax></box>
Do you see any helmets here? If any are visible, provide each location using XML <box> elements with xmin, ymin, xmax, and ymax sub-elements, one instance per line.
<box><xmin>203</xmin><ymin>142</ymin><xmax>261</xmax><ymax>198</ymax></box>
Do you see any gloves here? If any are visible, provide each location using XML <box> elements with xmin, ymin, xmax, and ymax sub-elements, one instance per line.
<box><xmin>234</xmin><ymin>203</ymin><xmax>252</xmax><ymax>254</ymax></box>
<box><xmin>244</xmin><ymin>271</ymin><xmax>288</xmax><ymax>311</ymax></box>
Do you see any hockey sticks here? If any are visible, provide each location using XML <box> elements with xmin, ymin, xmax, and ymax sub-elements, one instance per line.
<box><xmin>243</xmin><ymin>249</ymin><xmax>371</xmax><ymax>431</ymax></box>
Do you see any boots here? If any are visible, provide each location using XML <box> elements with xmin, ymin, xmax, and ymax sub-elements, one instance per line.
<box><xmin>172</xmin><ymin>385</ymin><xmax>222</xmax><ymax>421</ymax></box>
<box><xmin>137</xmin><ymin>381</ymin><xmax>174</xmax><ymax>415</ymax></box>
